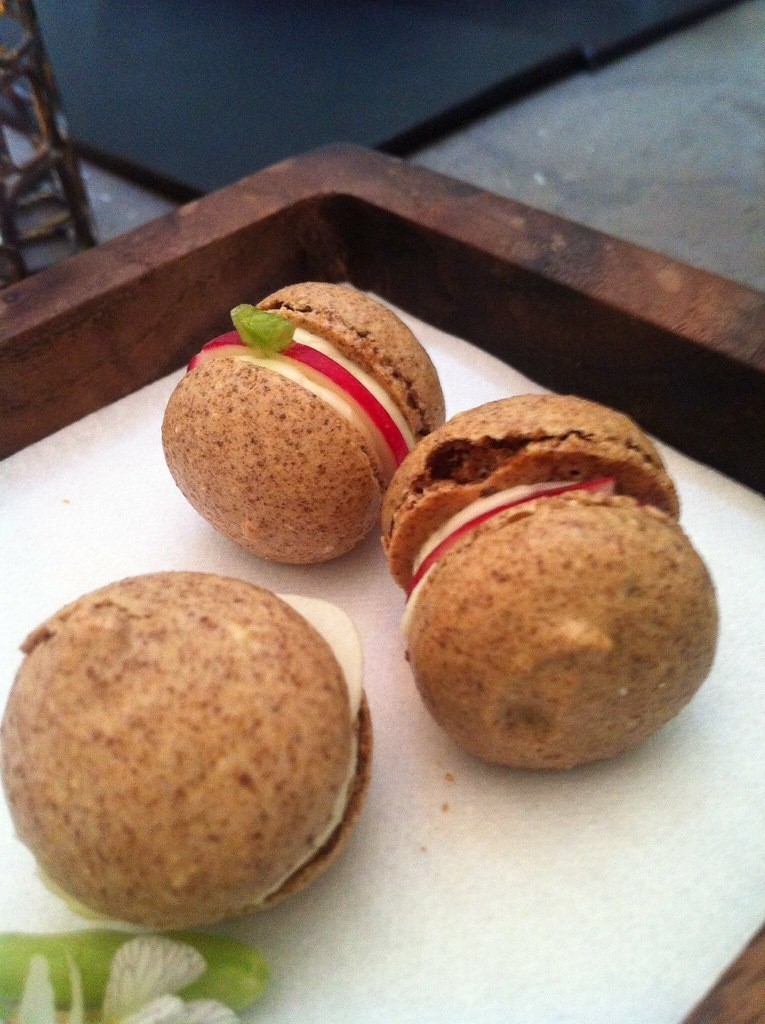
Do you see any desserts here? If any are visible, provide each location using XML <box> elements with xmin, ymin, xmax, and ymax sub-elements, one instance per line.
<box><xmin>161</xmin><ymin>280</ymin><xmax>448</xmax><ymax>565</ymax></box>
<box><xmin>0</xmin><ymin>569</ymin><xmax>376</xmax><ymax>929</ymax></box>
<box><xmin>379</xmin><ymin>395</ymin><xmax>722</xmax><ymax>772</ymax></box>
